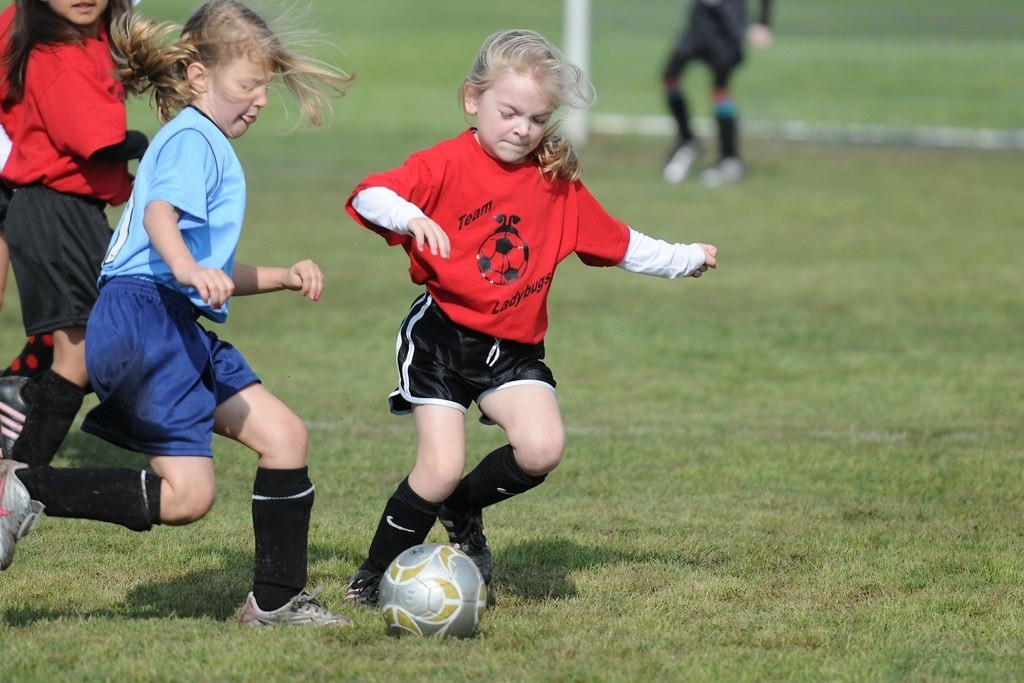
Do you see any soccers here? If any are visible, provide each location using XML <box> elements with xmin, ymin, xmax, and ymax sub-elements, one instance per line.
<box><xmin>378</xmin><ymin>542</ymin><xmax>488</xmax><ymax>639</ymax></box>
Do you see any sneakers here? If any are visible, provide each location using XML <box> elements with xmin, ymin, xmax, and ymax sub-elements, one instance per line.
<box><xmin>0</xmin><ymin>459</ymin><xmax>45</xmax><ymax>570</ymax></box>
<box><xmin>0</xmin><ymin>376</ymin><xmax>30</xmax><ymax>459</ymax></box>
<box><xmin>239</xmin><ymin>580</ymin><xmax>356</xmax><ymax>630</ymax></box>
<box><xmin>343</xmin><ymin>570</ymin><xmax>382</xmax><ymax>609</ymax></box>
<box><xmin>438</xmin><ymin>504</ymin><xmax>492</xmax><ymax>586</ymax></box>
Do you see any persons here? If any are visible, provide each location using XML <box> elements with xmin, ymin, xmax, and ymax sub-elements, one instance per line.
<box><xmin>0</xmin><ymin>0</ymin><xmax>354</xmax><ymax>630</ymax></box>
<box><xmin>660</xmin><ymin>0</ymin><xmax>773</xmax><ymax>185</ymax></box>
<box><xmin>345</xmin><ymin>31</ymin><xmax>717</xmax><ymax>606</ymax></box>
